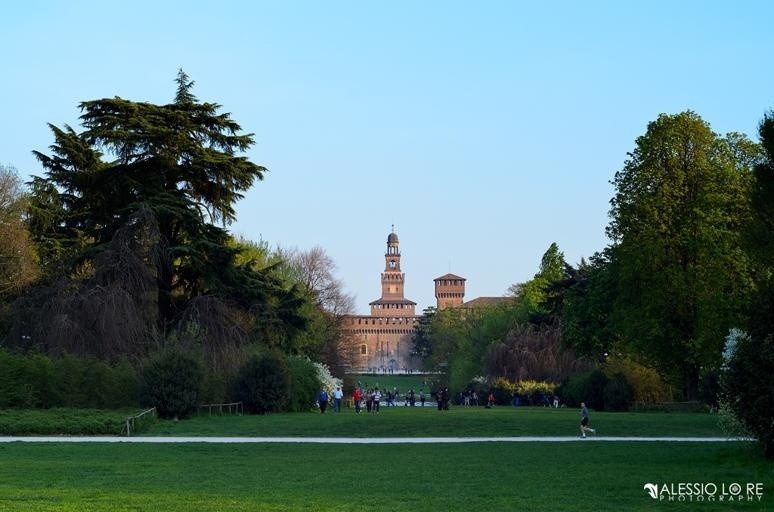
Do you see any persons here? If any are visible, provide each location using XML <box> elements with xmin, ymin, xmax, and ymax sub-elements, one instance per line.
<box><xmin>552</xmin><ymin>392</ymin><xmax>560</xmax><ymax>409</ymax></box>
<box><xmin>560</xmin><ymin>392</ymin><xmax>568</xmax><ymax>409</ymax></box>
<box><xmin>318</xmin><ymin>389</ymin><xmax>329</xmax><ymax>414</ymax></box>
<box><xmin>541</xmin><ymin>392</ymin><xmax>552</xmax><ymax>408</ymax></box>
<box><xmin>579</xmin><ymin>401</ymin><xmax>596</xmax><ymax>439</ymax></box>
<box><xmin>514</xmin><ymin>389</ymin><xmax>520</xmax><ymax>409</ymax></box>
<box><xmin>488</xmin><ymin>392</ymin><xmax>495</xmax><ymax>408</ymax></box>
<box><xmin>332</xmin><ymin>386</ymin><xmax>344</xmax><ymax>414</ymax></box>
<box><xmin>524</xmin><ymin>391</ymin><xmax>529</xmax><ymax>406</ymax></box>
<box><xmin>353</xmin><ymin>365</ymin><xmax>480</xmax><ymax>415</ymax></box>
